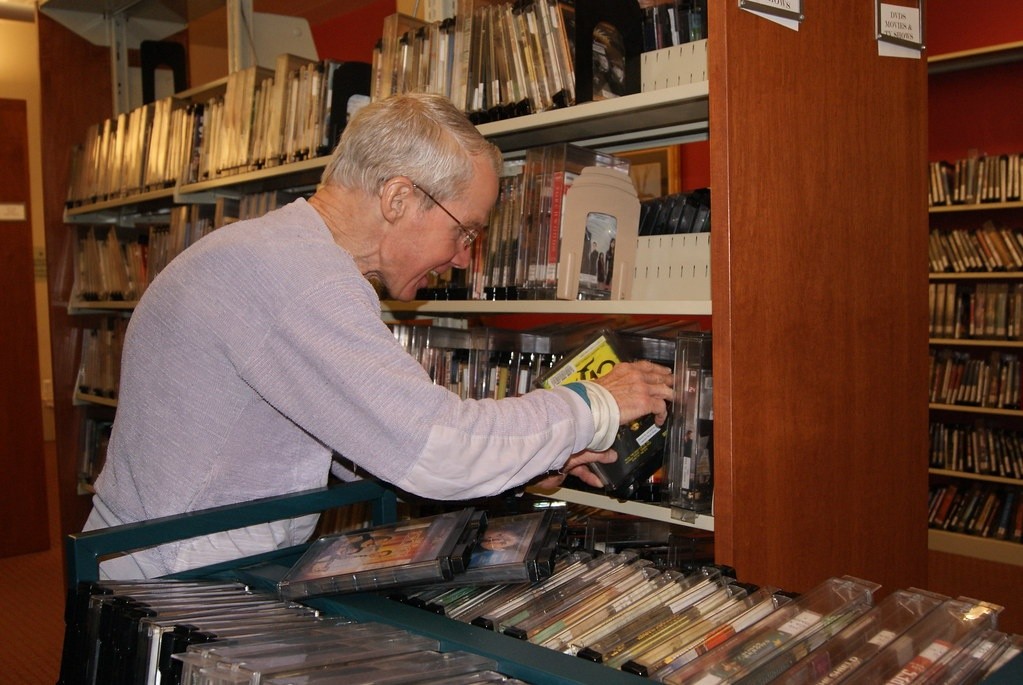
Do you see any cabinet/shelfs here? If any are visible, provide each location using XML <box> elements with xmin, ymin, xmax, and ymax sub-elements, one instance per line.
<box><xmin>65</xmin><ymin>476</ymin><xmax>671</xmax><ymax>685</ymax></box>
<box><xmin>68</xmin><ymin>1</ymin><xmax>931</xmax><ymax>596</ymax></box>
<box><xmin>922</xmin><ymin>0</ymin><xmax>1023</xmax><ymax>632</ymax></box>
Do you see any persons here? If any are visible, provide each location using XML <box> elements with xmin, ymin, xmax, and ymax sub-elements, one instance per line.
<box><xmin>479</xmin><ymin>525</ymin><xmax>524</xmax><ymax>552</ymax></box>
<box><xmin>318</xmin><ymin>534</ymin><xmax>391</xmax><ymax>570</ymax></box>
<box><xmin>83</xmin><ymin>94</ymin><xmax>676</xmax><ymax>578</ymax></box>
<box><xmin>582</xmin><ymin>214</ymin><xmax>615</xmax><ymax>287</ymax></box>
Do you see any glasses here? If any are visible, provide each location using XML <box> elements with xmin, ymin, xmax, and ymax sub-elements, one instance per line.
<box><xmin>414</xmin><ymin>181</ymin><xmax>484</xmax><ymax>250</ymax></box>
